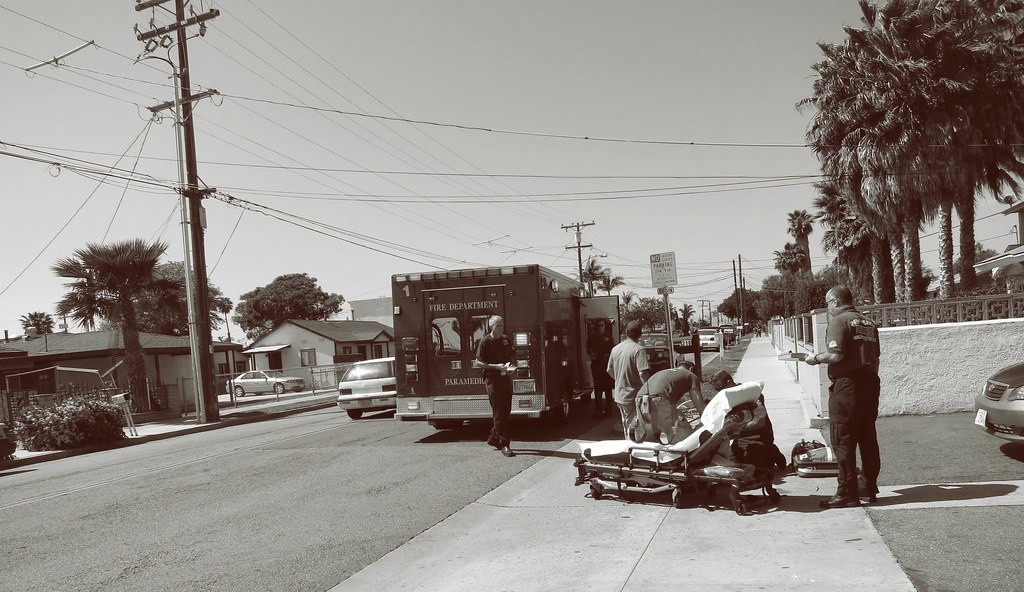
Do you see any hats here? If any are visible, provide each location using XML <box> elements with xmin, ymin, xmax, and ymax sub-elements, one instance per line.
<box><xmin>679</xmin><ymin>361</ymin><xmax>695</xmax><ymax>368</ymax></box>
<box><xmin>626</xmin><ymin>320</ymin><xmax>643</xmax><ymax>337</ymax></box>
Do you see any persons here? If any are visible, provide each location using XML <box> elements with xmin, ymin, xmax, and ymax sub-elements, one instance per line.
<box><xmin>753</xmin><ymin>322</ymin><xmax>763</xmax><ymax>338</ymax></box>
<box><xmin>635</xmin><ymin>360</ymin><xmax>706</xmax><ymax>444</ymax></box>
<box><xmin>700</xmin><ymin>370</ymin><xmax>786</xmax><ymax>470</ymax></box>
<box><xmin>606</xmin><ymin>320</ymin><xmax>651</xmax><ymax>440</ymax></box>
<box><xmin>476</xmin><ymin>316</ymin><xmax>517</xmax><ymax>457</ymax></box>
<box><xmin>805</xmin><ymin>285</ymin><xmax>880</xmax><ymax>508</ymax></box>
<box><xmin>586</xmin><ymin>319</ymin><xmax>614</xmax><ymax>419</ymax></box>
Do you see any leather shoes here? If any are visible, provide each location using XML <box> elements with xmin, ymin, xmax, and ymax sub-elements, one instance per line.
<box><xmin>502</xmin><ymin>446</ymin><xmax>516</xmax><ymax>457</ymax></box>
<box><xmin>487</xmin><ymin>440</ymin><xmax>502</xmax><ymax>450</ymax></box>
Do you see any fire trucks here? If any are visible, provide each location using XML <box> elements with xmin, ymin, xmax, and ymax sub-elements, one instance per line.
<box><xmin>390</xmin><ymin>263</ymin><xmax>622</xmax><ymax>431</ymax></box>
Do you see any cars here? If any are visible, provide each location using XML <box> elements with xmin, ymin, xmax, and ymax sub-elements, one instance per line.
<box><xmin>973</xmin><ymin>363</ymin><xmax>1024</xmax><ymax>442</ymax></box>
<box><xmin>637</xmin><ymin>322</ymin><xmax>752</xmax><ymax>376</ymax></box>
<box><xmin>337</xmin><ymin>357</ymin><xmax>396</xmax><ymax>420</ymax></box>
<box><xmin>225</xmin><ymin>369</ymin><xmax>305</xmax><ymax>395</ymax></box>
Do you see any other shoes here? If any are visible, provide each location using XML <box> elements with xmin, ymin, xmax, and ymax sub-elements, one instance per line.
<box><xmin>772</xmin><ymin>444</ymin><xmax>787</xmax><ymax>470</ymax></box>
<box><xmin>660</xmin><ymin>433</ymin><xmax>669</xmax><ymax>444</ymax></box>
<box><xmin>629</xmin><ymin>418</ymin><xmax>639</xmax><ymax>442</ymax></box>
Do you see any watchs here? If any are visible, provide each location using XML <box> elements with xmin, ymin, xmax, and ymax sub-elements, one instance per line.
<box><xmin>814</xmin><ymin>353</ymin><xmax>821</xmax><ymax>364</ymax></box>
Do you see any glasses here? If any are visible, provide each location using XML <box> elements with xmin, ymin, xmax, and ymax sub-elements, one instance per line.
<box><xmin>824</xmin><ymin>297</ymin><xmax>839</xmax><ymax>304</ymax></box>
<box><xmin>596</xmin><ymin>325</ymin><xmax>604</xmax><ymax>327</ymax></box>
<box><xmin>714</xmin><ymin>382</ymin><xmax>727</xmax><ymax>391</ymax></box>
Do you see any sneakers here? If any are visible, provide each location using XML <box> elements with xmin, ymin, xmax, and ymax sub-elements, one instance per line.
<box><xmin>859</xmin><ymin>495</ymin><xmax>877</xmax><ymax>502</ymax></box>
<box><xmin>819</xmin><ymin>492</ymin><xmax>861</xmax><ymax>508</ymax></box>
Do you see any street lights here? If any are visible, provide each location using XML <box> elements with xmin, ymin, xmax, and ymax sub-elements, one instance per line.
<box><xmin>132</xmin><ymin>53</ymin><xmax>208</xmax><ymax>426</ymax></box>
